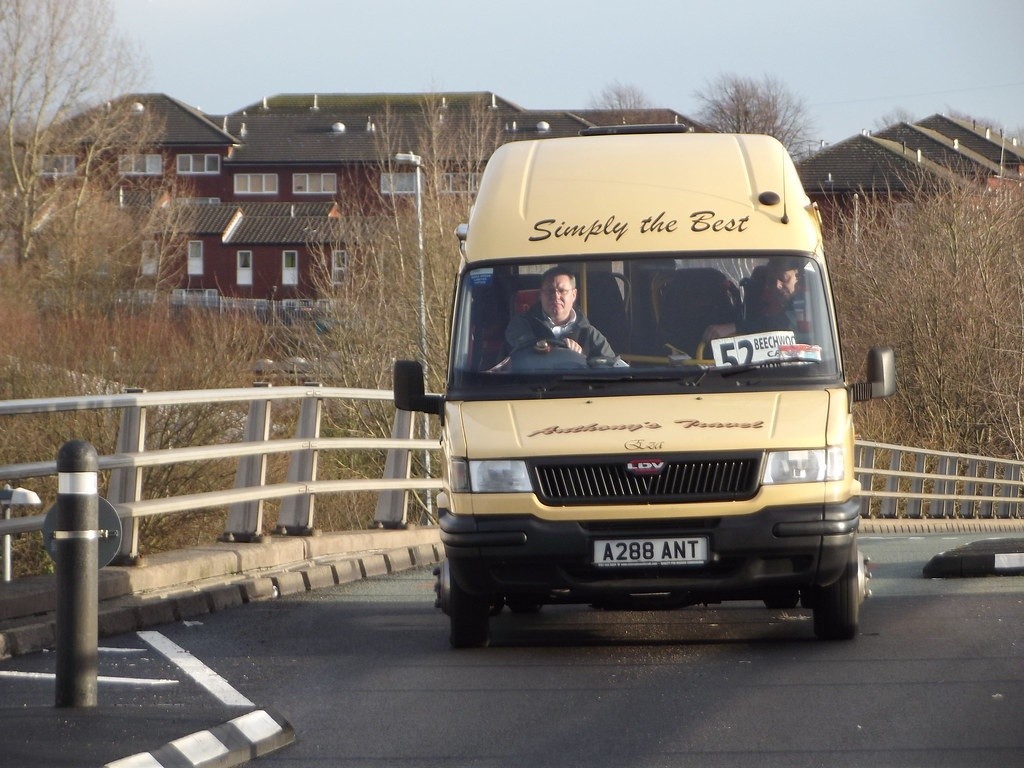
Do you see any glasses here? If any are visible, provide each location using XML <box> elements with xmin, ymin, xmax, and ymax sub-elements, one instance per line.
<box><xmin>541</xmin><ymin>286</ymin><xmax>574</xmax><ymax>297</ymax></box>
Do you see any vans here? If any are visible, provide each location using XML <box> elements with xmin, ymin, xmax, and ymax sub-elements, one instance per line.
<box><xmin>392</xmin><ymin>131</ymin><xmax>897</xmax><ymax>649</ymax></box>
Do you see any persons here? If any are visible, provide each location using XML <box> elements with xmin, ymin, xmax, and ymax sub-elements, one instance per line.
<box><xmin>505</xmin><ymin>267</ymin><xmax>616</xmax><ymax>368</ymax></box>
<box><xmin>703</xmin><ymin>259</ymin><xmax>806</xmax><ymax>345</ymax></box>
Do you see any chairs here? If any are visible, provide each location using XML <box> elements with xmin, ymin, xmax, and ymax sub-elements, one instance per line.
<box><xmin>511</xmin><ymin>266</ymin><xmax>764</xmax><ymax>357</ymax></box>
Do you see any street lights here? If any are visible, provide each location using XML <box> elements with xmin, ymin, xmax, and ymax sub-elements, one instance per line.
<box><xmin>395</xmin><ymin>149</ymin><xmax>435</xmax><ymax>524</ymax></box>
